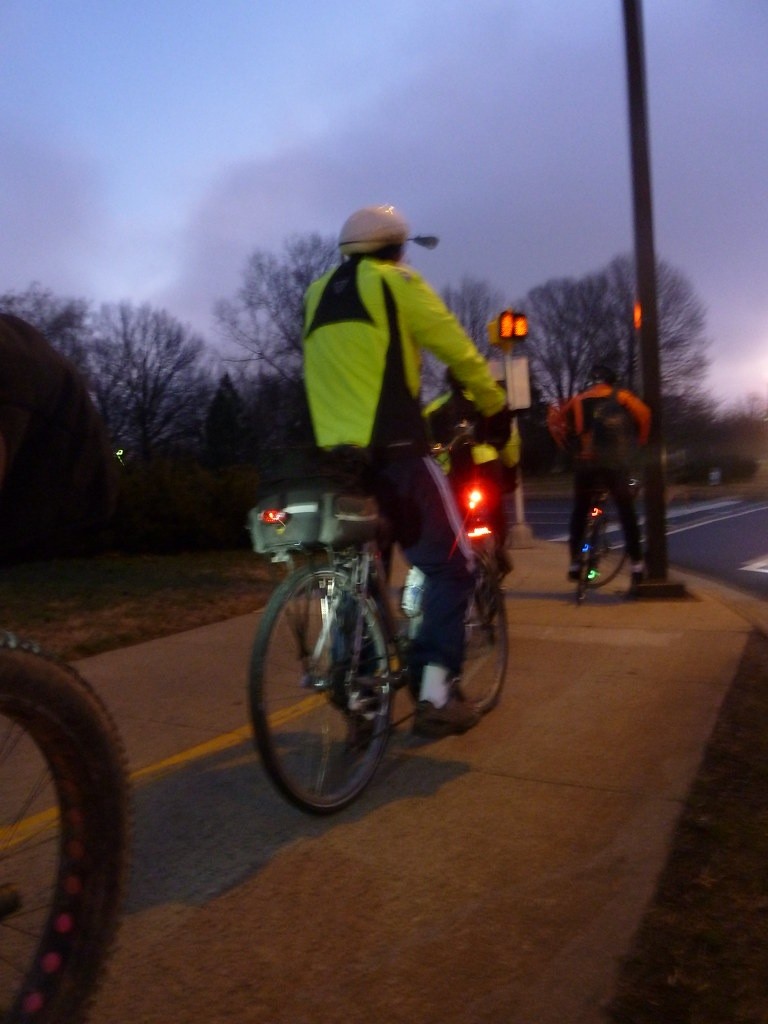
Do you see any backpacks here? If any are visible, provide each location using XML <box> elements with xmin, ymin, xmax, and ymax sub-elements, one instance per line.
<box><xmin>597</xmin><ymin>385</ymin><xmax>649</xmax><ymax>492</ymax></box>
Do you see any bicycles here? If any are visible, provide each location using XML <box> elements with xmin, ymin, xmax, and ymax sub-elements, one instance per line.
<box><xmin>1</xmin><ymin>643</ymin><xmax>132</xmax><ymax>1024</ymax></box>
<box><xmin>575</xmin><ymin>471</ymin><xmax>644</xmax><ymax>607</ymax></box>
<box><xmin>238</xmin><ymin>411</ymin><xmax>512</xmax><ymax>817</ymax></box>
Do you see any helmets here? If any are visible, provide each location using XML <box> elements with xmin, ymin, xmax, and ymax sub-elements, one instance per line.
<box><xmin>588</xmin><ymin>363</ymin><xmax>617</xmax><ymax>384</ymax></box>
<box><xmin>339</xmin><ymin>207</ymin><xmax>410</xmax><ymax>254</ymax></box>
<box><xmin>440</xmin><ymin>367</ymin><xmax>464</xmax><ymax>386</ymax></box>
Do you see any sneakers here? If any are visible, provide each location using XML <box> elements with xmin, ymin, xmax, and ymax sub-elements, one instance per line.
<box><xmin>628</xmin><ymin>572</ymin><xmax>645</xmax><ymax>583</ymax></box>
<box><xmin>567</xmin><ymin>566</ymin><xmax>585</xmax><ymax>582</ymax></box>
<box><xmin>418</xmin><ymin>697</ymin><xmax>477</xmax><ymax>739</ymax></box>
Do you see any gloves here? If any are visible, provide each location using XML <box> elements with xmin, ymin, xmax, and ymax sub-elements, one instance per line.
<box><xmin>473</xmin><ymin>404</ymin><xmax>513</xmax><ymax>439</ymax></box>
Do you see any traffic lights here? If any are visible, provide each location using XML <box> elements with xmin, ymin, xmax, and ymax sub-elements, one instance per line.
<box><xmin>497</xmin><ymin>309</ymin><xmax>531</xmax><ymax>344</ymax></box>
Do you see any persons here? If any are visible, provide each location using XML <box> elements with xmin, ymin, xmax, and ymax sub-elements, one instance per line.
<box><xmin>410</xmin><ymin>361</ymin><xmax>521</xmax><ymax>592</ymax></box>
<box><xmin>544</xmin><ymin>360</ymin><xmax>657</xmax><ymax>585</ymax></box>
<box><xmin>281</xmin><ymin>204</ymin><xmax>515</xmax><ymax>741</ymax></box>
